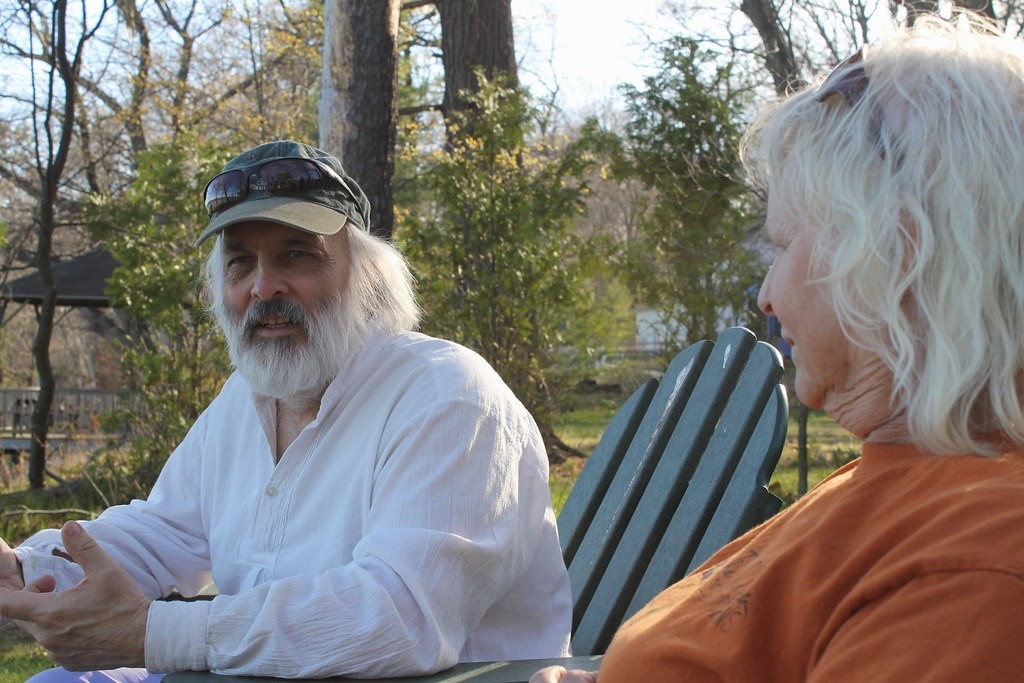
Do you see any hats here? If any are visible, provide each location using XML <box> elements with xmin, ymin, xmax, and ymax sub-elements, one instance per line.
<box><xmin>194</xmin><ymin>139</ymin><xmax>371</xmax><ymax>247</ymax></box>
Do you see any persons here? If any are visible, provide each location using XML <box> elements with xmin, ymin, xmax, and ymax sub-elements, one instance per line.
<box><xmin>0</xmin><ymin>140</ymin><xmax>573</xmax><ymax>683</ymax></box>
<box><xmin>526</xmin><ymin>9</ymin><xmax>1024</xmax><ymax>683</ymax></box>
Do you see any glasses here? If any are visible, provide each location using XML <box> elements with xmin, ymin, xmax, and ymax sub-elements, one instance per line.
<box><xmin>815</xmin><ymin>44</ymin><xmax>907</xmax><ymax>173</ymax></box>
<box><xmin>203</xmin><ymin>157</ymin><xmax>369</xmax><ymax>227</ymax></box>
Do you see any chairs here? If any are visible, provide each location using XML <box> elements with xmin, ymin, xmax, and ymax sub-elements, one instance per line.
<box><xmin>555</xmin><ymin>326</ymin><xmax>791</xmax><ymax>656</ymax></box>
